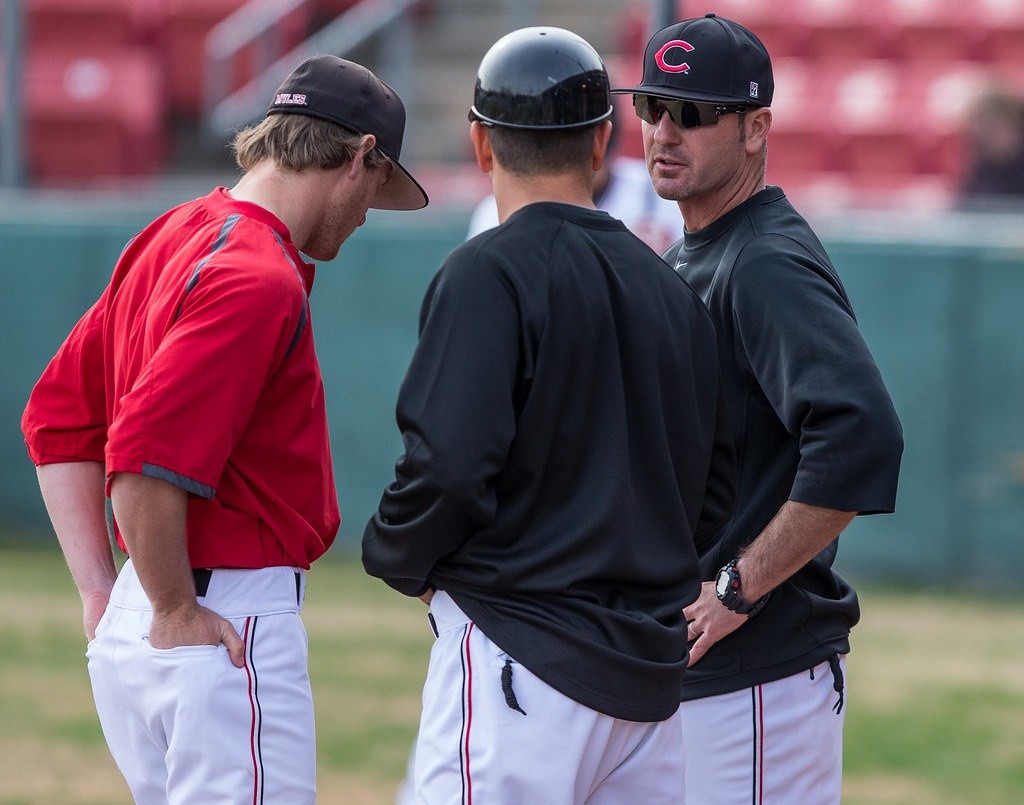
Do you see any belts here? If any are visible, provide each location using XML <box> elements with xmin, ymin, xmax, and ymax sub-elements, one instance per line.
<box><xmin>191</xmin><ymin>565</ymin><xmax>304</xmax><ymax>605</ymax></box>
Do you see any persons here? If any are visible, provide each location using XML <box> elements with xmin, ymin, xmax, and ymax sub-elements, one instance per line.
<box><xmin>397</xmin><ymin>85</ymin><xmax>684</xmax><ymax>805</ymax></box>
<box><xmin>610</xmin><ymin>14</ymin><xmax>904</xmax><ymax>805</ymax></box>
<box><xmin>951</xmin><ymin>91</ymin><xmax>1023</xmax><ymax>217</ymax></box>
<box><xmin>361</xmin><ymin>26</ymin><xmax>717</xmax><ymax>805</ymax></box>
<box><xmin>20</xmin><ymin>55</ymin><xmax>429</xmax><ymax>805</ymax></box>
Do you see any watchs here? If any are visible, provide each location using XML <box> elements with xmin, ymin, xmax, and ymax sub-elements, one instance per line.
<box><xmin>715</xmin><ymin>559</ymin><xmax>771</xmax><ymax>617</ymax></box>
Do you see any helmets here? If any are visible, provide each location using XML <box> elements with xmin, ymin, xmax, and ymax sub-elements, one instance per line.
<box><xmin>471</xmin><ymin>26</ymin><xmax>613</xmax><ymax>128</ymax></box>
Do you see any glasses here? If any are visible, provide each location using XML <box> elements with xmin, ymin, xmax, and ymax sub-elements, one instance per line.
<box><xmin>634</xmin><ymin>94</ymin><xmax>753</xmax><ymax>129</ymax></box>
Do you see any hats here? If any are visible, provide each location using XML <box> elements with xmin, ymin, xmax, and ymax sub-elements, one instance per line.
<box><xmin>611</xmin><ymin>14</ymin><xmax>774</xmax><ymax>107</ymax></box>
<box><xmin>267</xmin><ymin>54</ymin><xmax>429</xmax><ymax>211</ymax></box>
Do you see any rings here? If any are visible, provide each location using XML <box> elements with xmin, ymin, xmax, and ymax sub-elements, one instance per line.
<box><xmin>689</xmin><ymin>622</ymin><xmax>702</xmax><ymax>635</ymax></box>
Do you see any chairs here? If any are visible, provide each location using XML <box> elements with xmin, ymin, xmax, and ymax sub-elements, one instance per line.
<box><xmin>18</xmin><ymin>0</ymin><xmax>1024</xmax><ymax>208</ymax></box>
